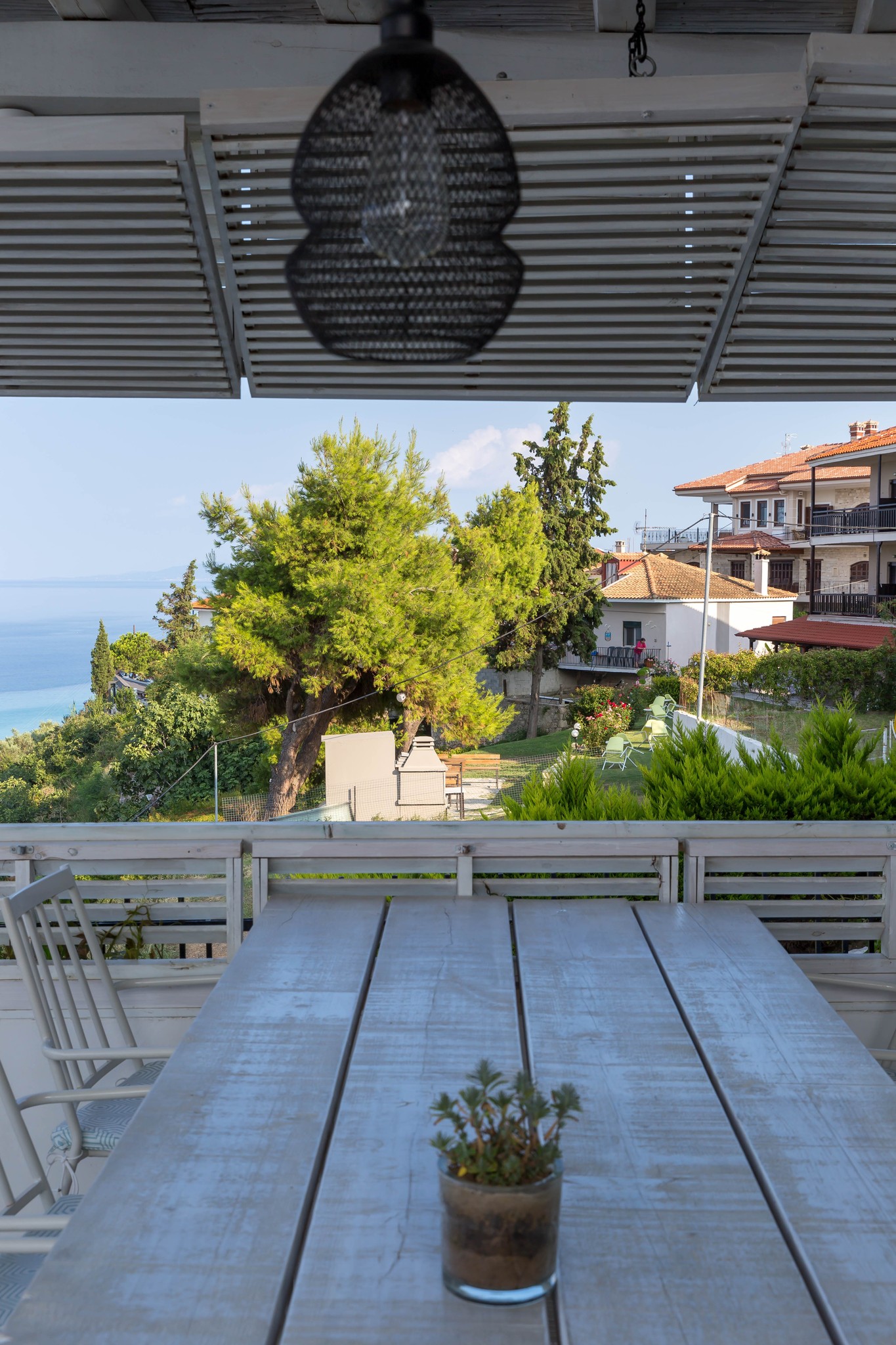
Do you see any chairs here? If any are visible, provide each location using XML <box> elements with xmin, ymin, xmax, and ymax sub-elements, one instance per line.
<box><xmin>0</xmin><ymin>1062</ymin><xmax>156</xmax><ymax>1324</ymax></box>
<box><xmin>0</xmin><ymin>863</ymin><xmax>171</xmax><ymax>1198</ymax></box>
<box><xmin>769</xmin><ymin>578</ymin><xmax>799</xmax><ymax>595</ymax></box>
<box><xmin>602</xmin><ymin>694</ymin><xmax>683</xmax><ymax>771</ymax></box>
<box><xmin>607</xmin><ymin>647</ymin><xmax>634</xmax><ymax>667</ymax></box>
<box><xmin>791</xmin><ymin>530</ymin><xmax>805</xmax><ymax>540</ymax></box>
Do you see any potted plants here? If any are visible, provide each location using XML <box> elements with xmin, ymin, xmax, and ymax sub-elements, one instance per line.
<box><xmin>429</xmin><ymin>1060</ymin><xmax>585</xmax><ymax>1304</ymax></box>
<box><xmin>680</xmin><ymin>536</ymin><xmax>687</xmax><ymax>541</ymax></box>
<box><xmin>642</xmin><ymin>652</ymin><xmax>657</xmax><ymax>667</ymax></box>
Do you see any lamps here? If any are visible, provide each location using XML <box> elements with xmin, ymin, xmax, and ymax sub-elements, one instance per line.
<box><xmin>785</xmin><ymin>512</ymin><xmax>786</xmax><ymax>516</ymax></box>
<box><xmin>893</xmin><ymin>472</ymin><xmax>896</xmax><ymax>476</ymax></box>
<box><xmin>769</xmin><ymin>513</ymin><xmax>771</xmax><ymax>516</ymax></box>
<box><xmin>286</xmin><ymin>0</ymin><xmax>526</xmax><ymax>362</ymax></box>
<box><xmin>752</xmin><ymin>513</ymin><xmax>753</xmax><ymax>517</ymax></box>
<box><xmin>571</xmin><ymin>723</ymin><xmax>581</xmax><ymax>751</ymax></box>
<box><xmin>396</xmin><ymin>692</ymin><xmax>406</xmax><ymax>702</ymax></box>
<box><xmin>736</xmin><ymin>514</ymin><xmax>738</xmax><ymax>517</ymax></box>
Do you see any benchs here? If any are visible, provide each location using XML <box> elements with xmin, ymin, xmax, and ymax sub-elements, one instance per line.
<box><xmin>438</xmin><ymin>752</ymin><xmax>501</xmax><ymax>810</ymax></box>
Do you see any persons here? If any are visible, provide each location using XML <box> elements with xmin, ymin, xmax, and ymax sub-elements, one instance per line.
<box><xmin>633</xmin><ymin>638</ymin><xmax>646</xmax><ymax>668</ymax></box>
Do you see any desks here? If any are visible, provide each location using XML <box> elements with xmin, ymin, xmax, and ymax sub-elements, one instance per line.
<box><xmin>445</xmin><ymin>787</ymin><xmax>467</xmax><ymax>820</ymax></box>
<box><xmin>605</xmin><ymin>741</ymin><xmax>630</xmax><ymax>769</ymax></box>
<box><xmin>664</xmin><ymin>699</ymin><xmax>671</xmax><ymax>713</ymax></box>
<box><xmin>644</xmin><ymin>708</ymin><xmax>653</xmax><ymax>720</ymax></box>
<box><xmin>591</xmin><ymin>654</ymin><xmax>605</xmax><ymax>666</ymax></box>
<box><xmin>0</xmin><ymin>891</ymin><xmax>896</xmax><ymax>1345</ymax></box>
<box><xmin>645</xmin><ymin>725</ymin><xmax>667</xmax><ymax>751</ymax></box>
